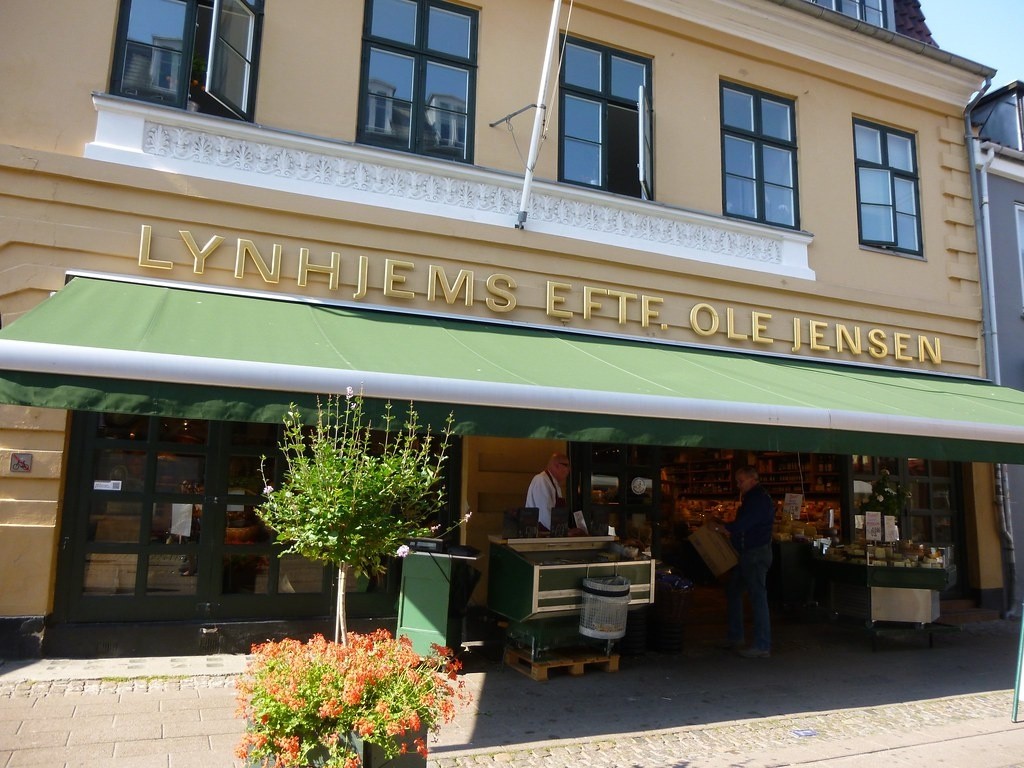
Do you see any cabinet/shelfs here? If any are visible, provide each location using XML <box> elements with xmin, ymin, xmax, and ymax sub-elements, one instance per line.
<box><xmin>396</xmin><ymin>535</ymin><xmax>655</xmax><ymax>662</ymax></box>
<box><xmin>668</xmin><ymin>448</ymin><xmax>844</xmax><ymax>498</ymax></box>
<box><xmin>773</xmin><ymin>542</ymin><xmax>957</xmax><ymax>624</ymax></box>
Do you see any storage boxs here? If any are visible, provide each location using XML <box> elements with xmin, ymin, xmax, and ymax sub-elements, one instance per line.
<box><xmin>688</xmin><ymin>524</ymin><xmax>740</xmax><ymax>577</ymax></box>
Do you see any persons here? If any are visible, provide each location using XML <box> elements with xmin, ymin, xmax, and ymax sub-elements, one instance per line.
<box><xmin>525</xmin><ymin>452</ymin><xmax>587</xmax><ymax>537</ymax></box>
<box><xmin>707</xmin><ymin>465</ymin><xmax>778</xmax><ymax>659</ymax></box>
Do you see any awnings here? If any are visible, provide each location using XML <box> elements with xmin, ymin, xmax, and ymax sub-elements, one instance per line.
<box><xmin>0</xmin><ymin>273</ymin><xmax>1024</xmax><ymax>464</ymax></box>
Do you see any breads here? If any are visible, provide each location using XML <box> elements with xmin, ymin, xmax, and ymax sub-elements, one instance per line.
<box><xmin>772</xmin><ymin>520</ymin><xmax>944</xmax><ymax>569</ymax></box>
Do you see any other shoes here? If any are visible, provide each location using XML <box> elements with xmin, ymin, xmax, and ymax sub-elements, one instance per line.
<box><xmin>736</xmin><ymin>648</ymin><xmax>770</xmax><ymax>658</ymax></box>
<box><xmin>717</xmin><ymin>639</ymin><xmax>745</xmax><ymax>650</ymax></box>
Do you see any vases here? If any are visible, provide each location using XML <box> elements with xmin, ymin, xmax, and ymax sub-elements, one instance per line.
<box><xmin>242</xmin><ymin>721</ymin><xmax>427</xmax><ymax>768</ymax></box>
<box><xmin>618</xmin><ymin>612</ymin><xmax>683</xmax><ymax>655</ymax></box>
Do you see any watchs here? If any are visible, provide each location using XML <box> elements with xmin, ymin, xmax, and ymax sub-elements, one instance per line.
<box><xmin>715</xmin><ymin>526</ymin><xmax>719</xmax><ymax>532</ymax></box>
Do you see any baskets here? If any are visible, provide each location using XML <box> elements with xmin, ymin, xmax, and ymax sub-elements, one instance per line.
<box><xmin>580</xmin><ymin>575</ymin><xmax>631</xmax><ymax>638</ymax></box>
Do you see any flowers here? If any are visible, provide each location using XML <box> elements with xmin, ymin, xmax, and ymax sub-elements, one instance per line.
<box><xmin>232</xmin><ymin>628</ymin><xmax>473</xmax><ymax>768</ymax></box>
<box><xmin>656</xmin><ymin>561</ymin><xmax>699</xmax><ymax>619</ymax></box>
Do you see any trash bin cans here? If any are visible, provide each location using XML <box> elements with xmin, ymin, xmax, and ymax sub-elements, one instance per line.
<box><xmin>579</xmin><ymin>576</ymin><xmax>631</xmax><ymax>640</ymax></box>
